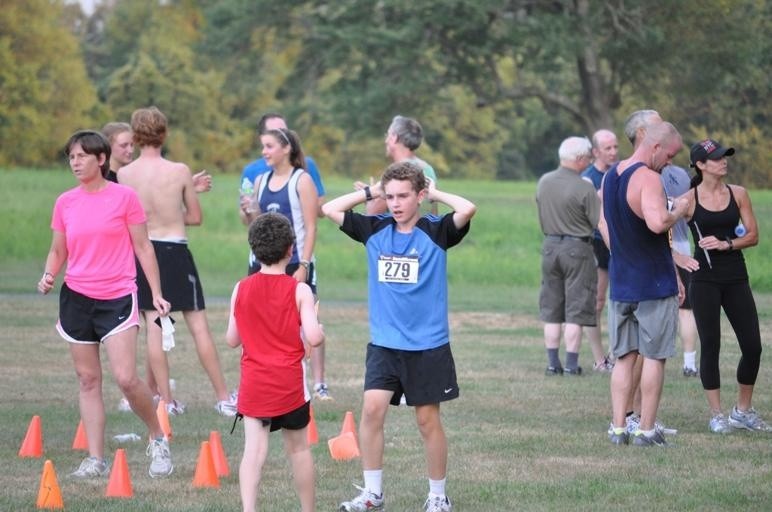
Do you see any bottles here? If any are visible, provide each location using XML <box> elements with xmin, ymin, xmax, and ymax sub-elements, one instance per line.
<box><xmin>169</xmin><ymin>377</ymin><xmax>176</xmax><ymax>400</ymax></box>
<box><xmin>241</xmin><ymin>177</ymin><xmax>259</xmax><ymax>214</ymax></box>
<box><xmin>734</xmin><ymin>224</ymin><xmax>746</xmax><ymax>238</ymax></box>
<box><xmin>108</xmin><ymin>433</ymin><xmax>142</xmax><ymax>450</ymax></box>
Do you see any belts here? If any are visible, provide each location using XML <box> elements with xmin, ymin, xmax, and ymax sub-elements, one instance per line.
<box><xmin>545</xmin><ymin>234</ymin><xmax>592</xmax><ymax>244</ymax></box>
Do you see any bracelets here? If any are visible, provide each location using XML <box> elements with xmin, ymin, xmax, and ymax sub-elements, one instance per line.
<box><xmin>43</xmin><ymin>272</ymin><xmax>55</xmax><ymax>279</ymax></box>
<box><xmin>726</xmin><ymin>236</ymin><xmax>732</xmax><ymax>251</ymax></box>
<box><xmin>299</xmin><ymin>260</ymin><xmax>311</xmax><ymax>272</ymax></box>
<box><xmin>364</xmin><ymin>185</ymin><xmax>372</xmax><ymax>201</ymax></box>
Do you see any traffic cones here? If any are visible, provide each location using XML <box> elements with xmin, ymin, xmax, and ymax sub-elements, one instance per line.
<box><xmin>307</xmin><ymin>405</ymin><xmax>320</xmax><ymax>447</ymax></box>
<box><xmin>16</xmin><ymin>415</ymin><xmax>47</xmax><ymax>457</ymax></box>
<box><xmin>103</xmin><ymin>449</ymin><xmax>137</xmax><ymax>498</ymax></box>
<box><xmin>327</xmin><ymin>432</ymin><xmax>358</xmax><ymax>462</ymax></box>
<box><xmin>339</xmin><ymin>409</ymin><xmax>357</xmax><ymax>436</ymax></box>
<box><xmin>150</xmin><ymin>398</ymin><xmax>174</xmax><ymax>443</ymax></box>
<box><xmin>70</xmin><ymin>420</ymin><xmax>90</xmax><ymax>450</ymax></box>
<box><xmin>208</xmin><ymin>431</ymin><xmax>230</xmax><ymax>477</ymax></box>
<box><xmin>37</xmin><ymin>456</ymin><xmax>65</xmax><ymax>510</ymax></box>
<box><xmin>191</xmin><ymin>439</ymin><xmax>221</xmax><ymax>488</ymax></box>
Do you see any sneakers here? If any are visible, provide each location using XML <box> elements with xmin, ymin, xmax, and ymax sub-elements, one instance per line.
<box><xmin>147</xmin><ymin>433</ymin><xmax>173</xmax><ymax>479</ymax></box>
<box><xmin>151</xmin><ymin>389</ymin><xmax>238</xmax><ymax>417</ymax></box>
<box><xmin>119</xmin><ymin>399</ymin><xmax>132</xmax><ymax>412</ymax></box>
<box><xmin>607</xmin><ymin>413</ymin><xmax>667</xmax><ymax>445</ymax></box>
<box><xmin>728</xmin><ymin>406</ymin><xmax>771</xmax><ymax>433</ymax></box>
<box><xmin>710</xmin><ymin>410</ymin><xmax>729</xmax><ymax>435</ymax></box>
<box><xmin>313</xmin><ymin>384</ymin><xmax>334</xmax><ymax>402</ymax></box>
<box><xmin>67</xmin><ymin>458</ymin><xmax>110</xmax><ymax>480</ymax></box>
<box><xmin>340</xmin><ymin>486</ymin><xmax>385</xmax><ymax>512</ymax></box>
<box><xmin>424</xmin><ymin>493</ymin><xmax>451</xmax><ymax>512</ymax></box>
<box><xmin>683</xmin><ymin>368</ymin><xmax>700</xmax><ymax>377</ymax></box>
<box><xmin>545</xmin><ymin>356</ymin><xmax>615</xmax><ymax>377</ymax></box>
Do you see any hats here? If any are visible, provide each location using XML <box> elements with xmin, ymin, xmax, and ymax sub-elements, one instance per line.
<box><xmin>690</xmin><ymin>139</ymin><xmax>736</xmax><ymax>165</ymax></box>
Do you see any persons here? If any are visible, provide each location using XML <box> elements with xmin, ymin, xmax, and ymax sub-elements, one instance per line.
<box><xmin>240</xmin><ymin>114</ymin><xmax>337</xmax><ymax>402</ymax></box>
<box><xmin>671</xmin><ymin>138</ymin><xmax>771</xmax><ymax>434</ymax></box>
<box><xmin>584</xmin><ymin>130</ymin><xmax>619</xmax><ymax>372</ymax></box>
<box><xmin>602</xmin><ymin>121</ymin><xmax>690</xmax><ymax>445</ymax></box>
<box><xmin>352</xmin><ymin>114</ymin><xmax>439</xmax><ymax>219</ymax></box>
<box><xmin>226</xmin><ymin>212</ymin><xmax>329</xmax><ymax>511</ymax></box>
<box><xmin>38</xmin><ymin>132</ymin><xmax>179</xmax><ymax>480</ymax></box>
<box><xmin>103</xmin><ymin>122</ymin><xmax>211</xmax><ymax>414</ymax></box>
<box><xmin>319</xmin><ymin>163</ymin><xmax>476</xmax><ymax>510</ymax></box>
<box><xmin>597</xmin><ymin>110</ymin><xmax>700</xmax><ymax>435</ymax></box>
<box><xmin>220</xmin><ymin>129</ymin><xmax>321</xmax><ymax>419</ymax></box>
<box><xmin>536</xmin><ymin>136</ymin><xmax>601</xmax><ymax>377</ymax></box>
<box><xmin>114</xmin><ymin>106</ymin><xmax>249</xmax><ymax>419</ymax></box>
